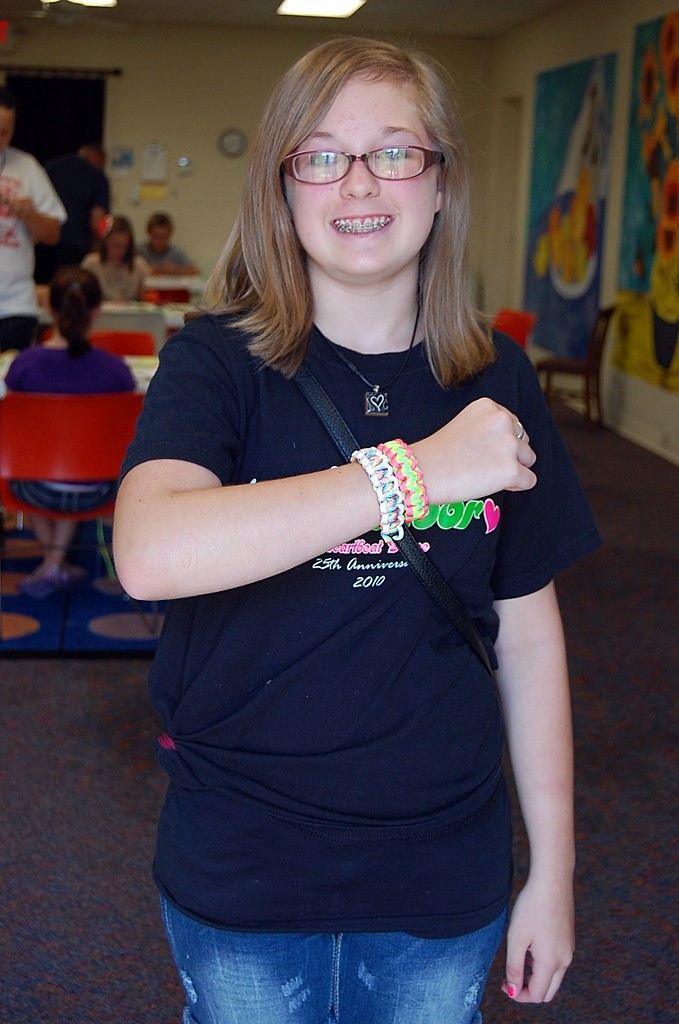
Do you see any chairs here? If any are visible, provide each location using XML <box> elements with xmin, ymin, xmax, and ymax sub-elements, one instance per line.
<box><xmin>492</xmin><ymin>309</ymin><xmax>538</xmax><ymax>350</ymax></box>
<box><xmin>143</xmin><ymin>289</ymin><xmax>190</xmax><ymax>306</ymax></box>
<box><xmin>0</xmin><ymin>389</ymin><xmax>145</xmax><ymax>574</ymax></box>
<box><xmin>533</xmin><ymin>303</ymin><xmax>617</xmax><ymax>430</ymax></box>
<box><xmin>93</xmin><ymin>310</ymin><xmax>167</xmax><ymax>354</ymax></box>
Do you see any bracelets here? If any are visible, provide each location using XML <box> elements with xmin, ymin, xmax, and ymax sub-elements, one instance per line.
<box><xmin>352</xmin><ymin>446</ymin><xmax>405</xmax><ymax>541</ymax></box>
<box><xmin>378</xmin><ymin>439</ymin><xmax>429</xmax><ymax>522</ymax></box>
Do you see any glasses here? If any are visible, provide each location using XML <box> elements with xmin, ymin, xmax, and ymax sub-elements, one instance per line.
<box><xmin>283</xmin><ymin>144</ymin><xmax>443</xmax><ymax>185</ymax></box>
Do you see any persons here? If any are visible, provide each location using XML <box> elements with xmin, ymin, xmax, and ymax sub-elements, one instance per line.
<box><xmin>0</xmin><ymin>87</ymin><xmax>70</xmax><ymax>378</ymax></box>
<box><xmin>108</xmin><ymin>33</ymin><xmax>596</xmax><ymax>1024</ymax></box>
<box><xmin>133</xmin><ymin>212</ymin><xmax>201</xmax><ymax>276</ymax></box>
<box><xmin>1</xmin><ymin>266</ymin><xmax>137</xmax><ymax>589</ymax></box>
<box><xmin>79</xmin><ymin>216</ymin><xmax>148</xmax><ymax>302</ymax></box>
<box><xmin>32</xmin><ymin>140</ymin><xmax>116</xmax><ymax>293</ymax></box>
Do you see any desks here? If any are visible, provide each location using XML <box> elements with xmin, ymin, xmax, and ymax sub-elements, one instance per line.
<box><xmin>145</xmin><ymin>273</ymin><xmax>210</xmax><ymax>306</ymax></box>
<box><xmin>0</xmin><ymin>350</ymin><xmax>159</xmax><ymax>396</ymax></box>
<box><xmin>34</xmin><ymin>304</ymin><xmax>205</xmax><ymax>349</ymax></box>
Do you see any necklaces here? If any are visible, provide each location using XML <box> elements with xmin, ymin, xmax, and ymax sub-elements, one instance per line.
<box><xmin>310</xmin><ymin>286</ymin><xmax>428</xmax><ymax>417</ymax></box>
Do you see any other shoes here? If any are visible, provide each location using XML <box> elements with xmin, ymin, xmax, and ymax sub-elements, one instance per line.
<box><xmin>16</xmin><ymin>564</ymin><xmax>61</xmax><ymax>599</ymax></box>
<box><xmin>61</xmin><ymin>567</ymin><xmax>79</xmax><ymax>586</ymax></box>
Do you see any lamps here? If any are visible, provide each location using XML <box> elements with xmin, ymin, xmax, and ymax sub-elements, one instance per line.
<box><xmin>217</xmin><ymin>127</ymin><xmax>247</xmax><ymax>159</ymax></box>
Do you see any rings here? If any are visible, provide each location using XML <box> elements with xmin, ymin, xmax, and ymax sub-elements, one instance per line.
<box><xmin>515</xmin><ymin>419</ymin><xmax>525</xmax><ymax>440</ymax></box>
